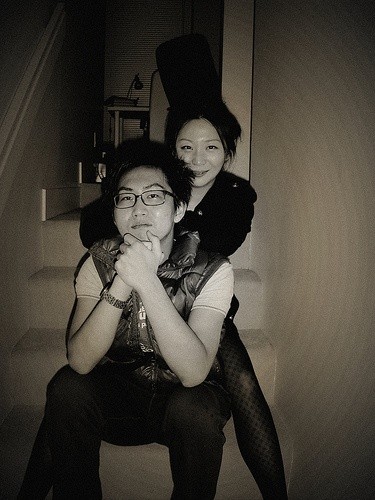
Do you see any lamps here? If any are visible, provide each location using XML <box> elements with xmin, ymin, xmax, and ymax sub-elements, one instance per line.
<box><xmin>127</xmin><ymin>71</ymin><xmax>145</xmax><ymax>99</ymax></box>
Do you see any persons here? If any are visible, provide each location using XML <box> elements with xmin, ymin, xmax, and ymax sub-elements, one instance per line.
<box><xmin>44</xmin><ymin>136</ymin><xmax>235</xmax><ymax>500</ymax></box>
<box><xmin>16</xmin><ymin>88</ymin><xmax>288</xmax><ymax>500</ymax></box>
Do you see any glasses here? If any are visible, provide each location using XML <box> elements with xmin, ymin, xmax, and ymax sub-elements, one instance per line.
<box><xmin>112</xmin><ymin>190</ymin><xmax>177</xmax><ymax>209</ymax></box>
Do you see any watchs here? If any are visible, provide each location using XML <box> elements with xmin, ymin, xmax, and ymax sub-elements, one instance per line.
<box><xmin>99</xmin><ymin>283</ymin><xmax>127</xmax><ymax>309</ymax></box>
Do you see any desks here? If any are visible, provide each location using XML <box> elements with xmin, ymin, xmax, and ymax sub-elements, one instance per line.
<box><xmin>106</xmin><ymin>105</ymin><xmax>149</xmax><ymax>152</ymax></box>
